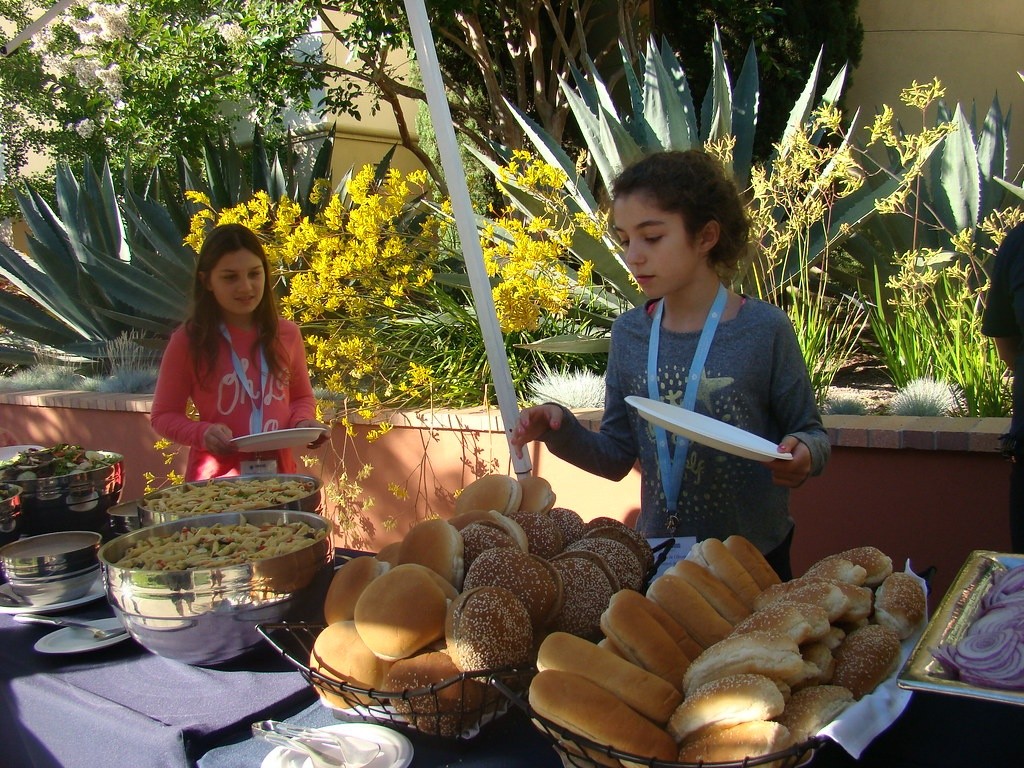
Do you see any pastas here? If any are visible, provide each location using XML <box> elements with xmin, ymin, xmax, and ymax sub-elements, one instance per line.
<box><xmin>139</xmin><ymin>478</ymin><xmax>317</xmax><ymax>514</ymax></box>
<box><xmin>112</xmin><ymin>510</ymin><xmax>326</xmax><ymax>571</ymax></box>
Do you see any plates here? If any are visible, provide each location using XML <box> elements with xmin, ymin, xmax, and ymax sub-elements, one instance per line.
<box><xmin>0</xmin><ymin>566</ymin><xmax>106</xmax><ymax>613</ymax></box>
<box><xmin>0</xmin><ymin>445</ymin><xmax>46</xmax><ymax>461</ymax></box>
<box><xmin>261</xmin><ymin>722</ymin><xmax>414</xmax><ymax>768</ymax></box>
<box><xmin>625</xmin><ymin>394</ymin><xmax>795</xmax><ymax>463</ymax></box>
<box><xmin>34</xmin><ymin>618</ymin><xmax>131</xmax><ymax>653</ymax></box>
<box><xmin>230</xmin><ymin>426</ymin><xmax>326</xmax><ymax>452</ymax></box>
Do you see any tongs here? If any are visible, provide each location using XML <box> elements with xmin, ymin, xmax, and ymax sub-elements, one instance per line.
<box><xmin>252</xmin><ymin>720</ymin><xmax>381</xmax><ymax>768</ymax></box>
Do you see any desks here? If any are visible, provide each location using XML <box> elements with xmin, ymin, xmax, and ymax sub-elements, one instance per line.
<box><xmin>0</xmin><ymin>605</ymin><xmax>564</xmax><ymax>767</ymax></box>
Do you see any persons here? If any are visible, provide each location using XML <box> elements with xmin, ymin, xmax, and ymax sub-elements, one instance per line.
<box><xmin>510</xmin><ymin>148</ymin><xmax>830</xmax><ymax>585</ymax></box>
<box><xmin>152</xmin><ymin>224</ymin><xmax>331</xmax><ymax>482</ymax></box>
<box><xmin>983</xmin><ymin>219</ymin><xmax>1024</xmax><ymax>564</ymax></box>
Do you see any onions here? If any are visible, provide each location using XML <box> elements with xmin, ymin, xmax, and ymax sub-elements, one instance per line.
<box><xmin>929</xmin><ymin>562</ymin><xmax>1024</xmax><ymax>692</ymax></box>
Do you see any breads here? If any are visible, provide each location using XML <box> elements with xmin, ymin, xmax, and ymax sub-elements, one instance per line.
<box><xmin>663</xmin><ymin>544</ymin><xmax>926</xmax><ymax>768</ymax></box>
<box><xmin>308</xmin><ymin>474</ymin><xmax>654</xmax><ymax>736</ymax></box>
<box><xmin>528</xmin><ymin>535</ymin><xmax>785</xmax><ymax>768</ymax></box>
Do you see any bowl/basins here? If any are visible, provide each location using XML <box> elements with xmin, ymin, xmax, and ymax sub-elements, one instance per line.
<box><xmin>0</xmin><ymin>483</ymin><xmax>23</xmax><ymax>544</ymax></box>
<box><xmin>0</xmin><ymin>450</ymin><xmax>125</xmax><ymax>530</ymax></box>
<box><xmin>107</xmin><ymin>501</ymin><xmax>142</xmax><ymax>532</ymax></box>
<box><xmin>333</xmin><ymin>554</ymin><xmax>352</xmax><ymax>572</ymax></box>
<box><xmin>0</xmin><ymin>532</ymin><xmax>102</xmax><ymax>604</ymax></box>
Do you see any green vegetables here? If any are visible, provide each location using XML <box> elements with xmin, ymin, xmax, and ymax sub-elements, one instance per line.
<box><xmin>0</xmin><ymin>442</ymin><xmax>123</xmax><ymax>501</ymax></box>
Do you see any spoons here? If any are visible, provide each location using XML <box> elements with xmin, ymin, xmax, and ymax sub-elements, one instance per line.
<box><xmin>0</xmin><ymin>593</ymin><xmax>19</xmax><ymax>607</ymax></box>
<box><xmin>14</xmin><ymin>614</ymin><xmax>126</xmax><ymax>639</ymax></box>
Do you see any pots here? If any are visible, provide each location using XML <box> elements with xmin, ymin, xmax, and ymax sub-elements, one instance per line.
<box><xmin>96</xmin><ymin>509</ymin><xmax>335</xmax><ymax>663</ymax></box>
<box><xmin>139</xmin><ymin>475</ymin><xmax>325</xmax><ymax>530</ymax></box>
